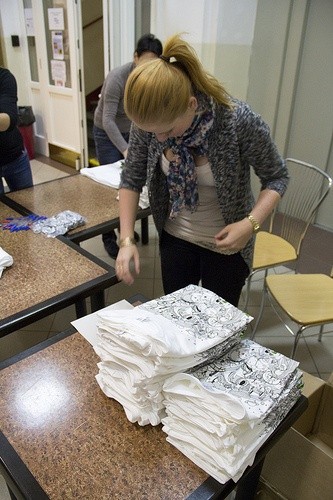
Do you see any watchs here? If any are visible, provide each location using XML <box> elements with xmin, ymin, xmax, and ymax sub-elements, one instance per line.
<box><xmin>246</xmin><ymin>213</ymin><xmax>263</xmax><ymax>235</ymax></box>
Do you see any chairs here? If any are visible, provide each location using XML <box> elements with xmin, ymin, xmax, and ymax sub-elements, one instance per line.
<box><xmin>244</xmin><ymin>158</ymin><xmax>333</xmax><ymax>434</ymax></box>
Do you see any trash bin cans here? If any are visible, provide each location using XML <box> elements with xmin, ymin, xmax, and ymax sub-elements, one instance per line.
<box><xmin>17</xmin><ymin>105</ymin><xmax>35</xmax><ymax>159</ymax></box>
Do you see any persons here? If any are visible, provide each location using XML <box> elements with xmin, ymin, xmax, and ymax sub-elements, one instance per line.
<box><xmin>92</xmin><ymin>33</ymin><xmax>163</xmax><ymax>260</ymax></box>
<box><xmin>114</xmin><ymin>38</ymin><xmax>290</xmax><ymax>336</ymax></box>
<box><xmin>0</xmin><ymin>68</ymin><xmax>34</xmax><ymax>196</ymax></box>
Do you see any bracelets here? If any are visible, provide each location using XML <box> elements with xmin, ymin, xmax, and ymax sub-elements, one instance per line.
<box><xmin>116</xmin><ymin>236</ymin><xmax>137</xmax><ymax>247</ymax></box>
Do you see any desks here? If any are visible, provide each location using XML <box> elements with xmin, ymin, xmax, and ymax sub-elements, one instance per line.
<box><xmin>0</xmin><ymin>172</ymin><xmax>152</xmax><ymax>336</ymax></box>
<box><xmin>0</xmin><ymin>292</ymin><xmax>307</xmax><ymax>500</ymax></box>
